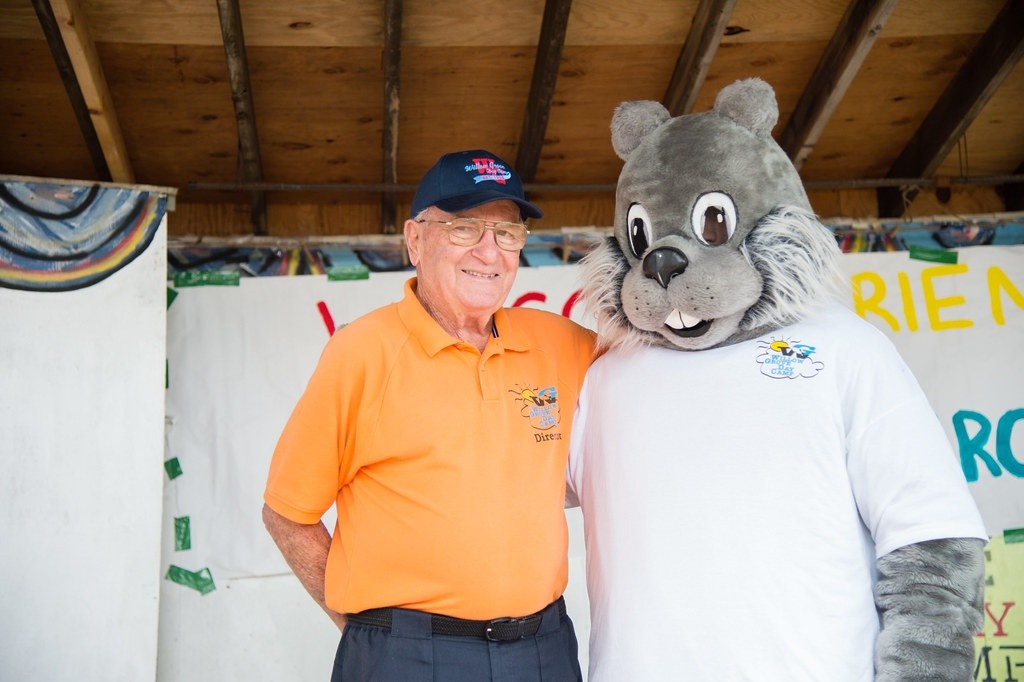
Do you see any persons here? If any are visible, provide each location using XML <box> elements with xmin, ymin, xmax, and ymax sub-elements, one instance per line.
<box><xmin>260</xmin><ymin>149</ymin><xmax>608</xmax><ymax>682</ymax></box>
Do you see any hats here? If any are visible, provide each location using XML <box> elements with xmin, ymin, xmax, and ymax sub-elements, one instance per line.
<box><xmin>412</xmin><ymin>151</ymin><xmax>545</xmax><ymax>222</ymax></box>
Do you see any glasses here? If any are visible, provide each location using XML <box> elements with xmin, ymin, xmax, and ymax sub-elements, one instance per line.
<box><xmin>416</xmin><ymin>215</ymin><xmax>529</xmax><ymax>252</ymax></box>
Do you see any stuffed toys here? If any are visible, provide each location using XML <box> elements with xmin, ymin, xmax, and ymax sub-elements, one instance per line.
<box><xmin>564</xmin><ymin>78</ymin><xmax>986</xmax><ymax>681</ymax></box>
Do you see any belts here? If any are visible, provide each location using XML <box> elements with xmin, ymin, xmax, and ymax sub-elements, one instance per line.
<box><xmin>349</xmin><ymin>599</ymin><xmax>565</xmax><ymax>643</ymax></box>
